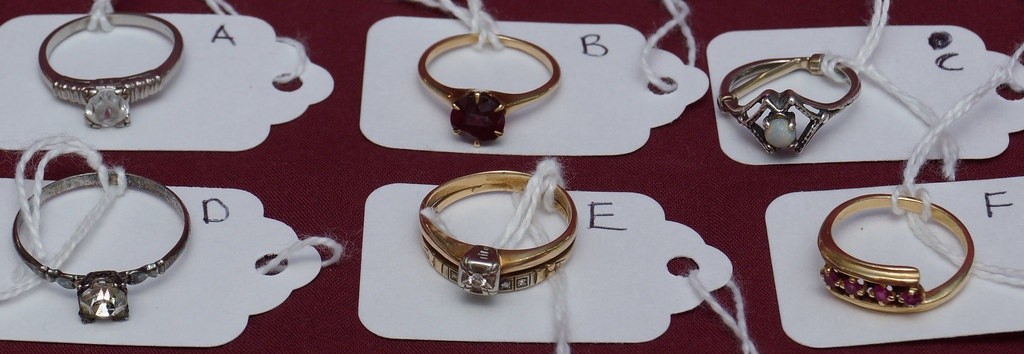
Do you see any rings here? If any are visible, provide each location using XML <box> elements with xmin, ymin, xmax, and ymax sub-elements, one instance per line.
<box><xmin>417</xmin><ymin>170</ymin><xmax>578</xmax><ymax>297</ymax></box>
<box><xmin>38</xmin><ymin>10</ymin><xmax>185</xmax><ymax>130</ymax></box>
<box><xmin>717</xmin><ymin>54</ymin><xmax>863</xmax><ymax>155</ymax></box>
<box><xmin>12</xmin><ymin>171</ymin><xmax>193</xmax><ymax>325</ymax></box>
<box><xmin>417</xmin><ymin>33</ymin><xmax>562</xmax><ymax>149</ymax></box>
<box><xmin>817</xmin><ymin>193</ymin><xmax>975</xmax><ymax>314</ymax></box>
